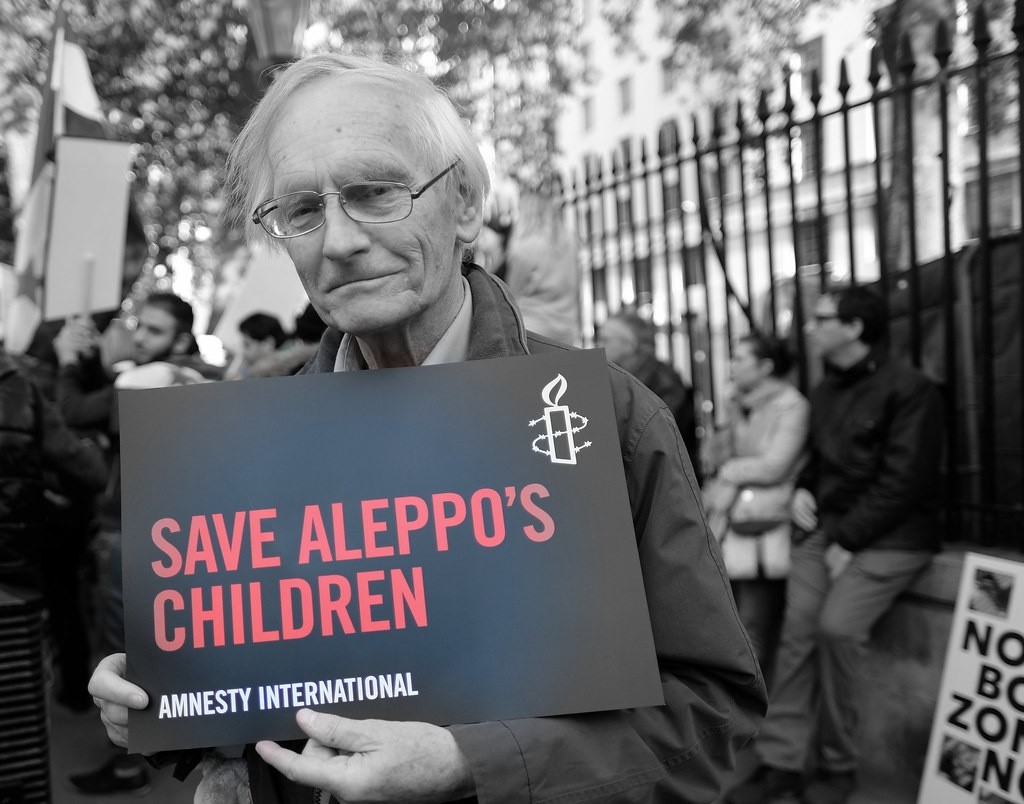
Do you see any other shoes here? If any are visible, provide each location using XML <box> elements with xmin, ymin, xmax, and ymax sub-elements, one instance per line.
<box><xmin>70</xmin><ymin>760</ymin><xmax>152</xmax><ymax>796</ymax></box>
<box><xmin>724</xmin><ymin>767</ymin><xmax>813</xmax><ymax>804</ymax></box>
<box><xmin>798</xmin><ymin>768</ymin><xmax>860</xmax><ymax>803</ymax></box>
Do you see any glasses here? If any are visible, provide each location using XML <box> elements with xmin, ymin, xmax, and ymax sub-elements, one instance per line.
<box><xmin>251</xmin><ymin>158</ymin><xmax>464</xmax><ymax>237</ymax></box>
<box><xmin>815</xmin><ymin>315</ymin><xmax>841</xmax><ymax>325</ymax></box>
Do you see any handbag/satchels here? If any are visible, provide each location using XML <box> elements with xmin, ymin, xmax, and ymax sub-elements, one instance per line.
<box><xmin>729</xmin><ymin>481</ymin><xmax>796</xmax><ymax>535</ymax></box>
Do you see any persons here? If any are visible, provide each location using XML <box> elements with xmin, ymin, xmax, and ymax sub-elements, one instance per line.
<box><xmin>87</xmin><ymin>53</ymin><xmax>767</xmax><ymax>804</ymax></box>
<box><xmin>591</xmin><ymin>287</ymin><xmax>961</xmax><ymax>804</ymax></box>
<box><xmin>0</xmin><ymin>294</ymin><xmax>331</xmax><ymax>804</ymax></box>
